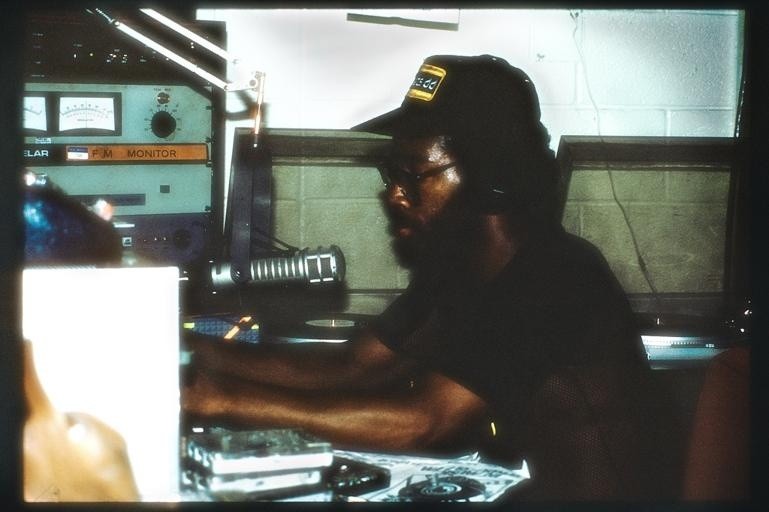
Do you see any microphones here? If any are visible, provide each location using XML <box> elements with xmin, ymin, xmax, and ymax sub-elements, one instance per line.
<box><xmin>210</xmin><ymin>244</ymin><xmax>345</xmax><ymax>291</ymax></box>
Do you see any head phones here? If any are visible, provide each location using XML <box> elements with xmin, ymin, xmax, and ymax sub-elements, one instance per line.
<box><xmin>461</xmin><ymin>58</ymin><xmax>524</xmax><ymax>218</ymax></box>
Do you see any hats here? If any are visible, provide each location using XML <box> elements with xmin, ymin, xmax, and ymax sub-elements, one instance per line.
<box><xmin>348</xmin><ymin>54</ymin><xmax>543</xmax><ymax>140</ymax></box>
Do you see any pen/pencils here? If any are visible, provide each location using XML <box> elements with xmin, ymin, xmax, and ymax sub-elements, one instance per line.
<box><xmin>672</xmin><ymin>342</ymin><xmax>735</xmax><ymax>351</ymax></box>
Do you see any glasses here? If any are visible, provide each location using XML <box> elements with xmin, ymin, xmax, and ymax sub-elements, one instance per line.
<box><xmin>376</xmin><ymin>152</ymin><xmax>460</xmax><ymax>204</ymax></box>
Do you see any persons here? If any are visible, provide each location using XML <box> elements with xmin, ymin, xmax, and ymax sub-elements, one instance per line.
<box><xmin>180</xmin><ymin>55</ymin><xmax>662</xmax><ymax>512</ymax></box>
